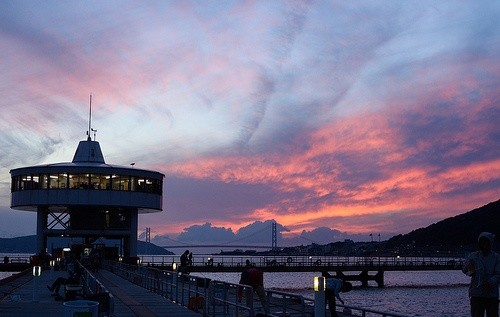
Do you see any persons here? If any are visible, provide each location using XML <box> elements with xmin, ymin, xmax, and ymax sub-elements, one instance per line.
<box><xmin>180</xmin><ymin>250</ymin><xmax>190</xmax><ymax>274</ymax></box>
<box><xmin>325</xmin><ymin>278</ymin><xmax>352</xmax><ymax>317</ymax></box>
<box><xmin>47</xmin><ymin>264</ymin><xmax>81</xmax><ymax>296</ymax></box>
<box><xmin>54</xmin><ymin>248</ymin><xmax>62</xmax><ymax>263</ymax></box>
<box><xmin>91</xmin><ymin>251</ymin><xmax>100</xmax><ymax>273</ymax></box>
<box><xmin>462</xmin><ymin>232</ymin><xmax>500</xmax><ymax>317</ymax></box>
<box><xmin>237</xmin><ymin>260</ymin><xmax>270</xmax><ymax>317</ymax></box>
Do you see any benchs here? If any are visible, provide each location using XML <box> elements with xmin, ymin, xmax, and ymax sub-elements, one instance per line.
<box><xmin>64</xmin><ymin>282</ymin><xmax>84</xmax><ymax>298</ymax></box>
